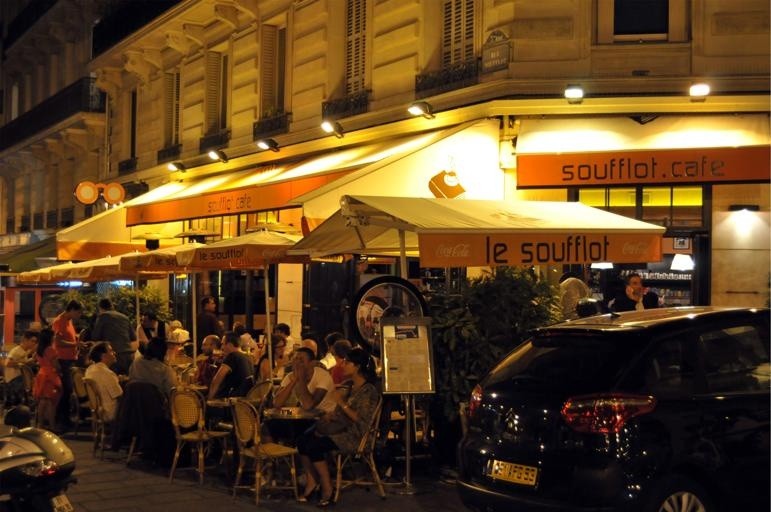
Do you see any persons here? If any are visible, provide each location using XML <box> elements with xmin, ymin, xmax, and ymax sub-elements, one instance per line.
<box><xmin>612</xmin><ymin>274</ymin><xmax>658</xmax><ymax>312</ymax></box>
<box><xmin>3</xmin><ymin>296</ymin><xmax>378</xmax><ymax>508</ymax></box>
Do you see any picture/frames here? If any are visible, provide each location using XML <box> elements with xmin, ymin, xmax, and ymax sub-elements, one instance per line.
<box><xmin>672</xmin><ymin>236</ymin><xmax>689</xmax><ymax>250</ymax></box>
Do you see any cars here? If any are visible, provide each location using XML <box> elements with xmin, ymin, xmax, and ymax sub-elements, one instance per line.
<box><xmin>373</xmin><ymin>366</ymin><xmax>425</xmax><ymax>445</ymax></box>
<box><xmin>457</xmin><ymin>305</ymin><xmax>771</xmax><ymax>511</ymax></box>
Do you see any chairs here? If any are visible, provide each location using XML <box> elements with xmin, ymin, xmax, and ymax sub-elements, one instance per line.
<box><xmin>0</xmin><ymin>350</ymin><xmax>434</xmax><ymax>507</ymax></box>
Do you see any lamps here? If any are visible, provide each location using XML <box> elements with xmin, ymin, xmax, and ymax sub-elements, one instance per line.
<box><xmin>258</xmin><ymin>138</ymin><xmax>279</xmax><ymax>153</ymax></box>
<box><xmin>565</xmin><ymin>83</ymin><xmax>584</xmax><ymax>105</ymax></box>
<box><xmin>165</xmin><ymin>156</ymin><xmax>187</xmax><ymax>178</ymax></box>
<box><xmin>203</xmin><ymin>150</ymin><xmax>229</xmax><ymax>163</ymax></box>
<box><xmin>688</xmin><ymin>78</ymin><xmax>711</xmax><ymax>103</ymax></box>
<box><xmin>409</xmin><ymin>99</ymin><xmax>437</xmax><ymax>123</ymax></box>
<box><xmin>320</xmin><ymin>118</ymin><xmax>348</xmax><ymax>142</ymax></box>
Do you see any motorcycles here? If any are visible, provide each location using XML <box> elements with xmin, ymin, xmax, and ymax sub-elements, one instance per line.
<box><xmin>0</xmin><ymin>406</ymin><xmax>77</xmax><ymax>512</ymax></box>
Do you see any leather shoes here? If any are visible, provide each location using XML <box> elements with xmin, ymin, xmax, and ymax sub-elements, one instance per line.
<box><xmin>297</xmin><ymin>486</ymin><xmax>319</xmax><ymax>503</ymax></box>
<box><xmin>320</xmin><ymin>488</ymin><xmax>337</xmax><ymax>507</ymax></box>
